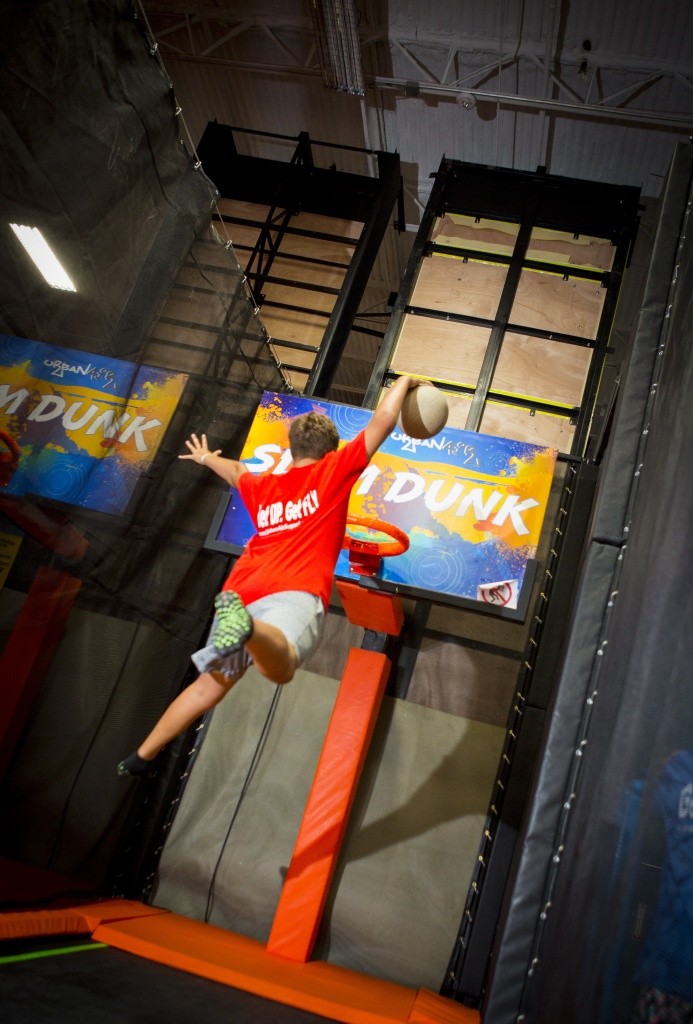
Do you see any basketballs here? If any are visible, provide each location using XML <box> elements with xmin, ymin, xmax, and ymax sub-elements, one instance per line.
<box><xmin>396</xmin><ymin>385</ymin><xmax>449</xmax><ymax>440</ymax></box>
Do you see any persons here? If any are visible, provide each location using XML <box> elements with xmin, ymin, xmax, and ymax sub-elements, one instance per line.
<box><xmin>114</xmin><ymin>373</ymin><xmax>441</xmax><ymax>790</ymax></box>
